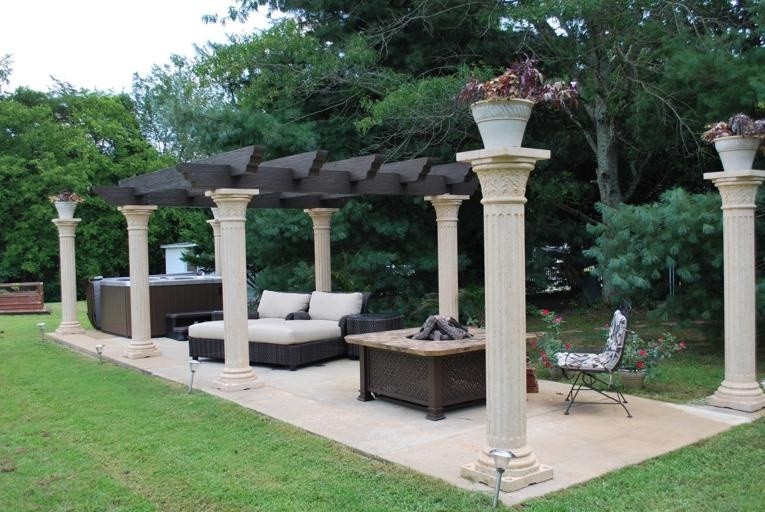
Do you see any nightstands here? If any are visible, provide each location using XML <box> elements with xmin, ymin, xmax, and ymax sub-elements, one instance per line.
<box><xmin>338</xmin><ymin>313</ymin><xmax>405</xmax><ymax>360</ymax></box>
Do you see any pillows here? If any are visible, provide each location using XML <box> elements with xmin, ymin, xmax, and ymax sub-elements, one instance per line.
<box><xmin>257</xmin><ymin>288</ymin><xmax>372</xmax><ymax>321</ymax></box>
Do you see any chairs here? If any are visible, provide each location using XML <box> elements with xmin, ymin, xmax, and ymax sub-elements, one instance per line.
<box><xmin>554</xmin><ymin>310</ymin><xmax>633</xmax><ymax>418</ymax></box>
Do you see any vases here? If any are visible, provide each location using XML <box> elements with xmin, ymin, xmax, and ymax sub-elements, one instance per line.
<box><xmin>712</xmin><ymin>134</ymin><xmax>764</xmax><ymax>171</ymax></box>
<box><xmin>614</xmin><ymin>366</ymin><xmax>647</xmax><ymax>390</ymax></box>
<box><xmin>549</xmin><ymin>367</ymin><xmax>563</xmax><ymax>380</ymax></box>
<box><xmin>469</xmin><ymin>97</ymin><xmax>536</xmax><ymax>149</ymax></box>
<box><xmin>53</xmin><ymin>201</ymin><xmax>79</xmax><ymax>219</ymax></box>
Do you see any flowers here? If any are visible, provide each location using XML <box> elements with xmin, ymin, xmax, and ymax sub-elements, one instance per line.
<box><xmin>529</xmin><ymin>307</ymin><xmax>575</xmax><ymax>368</ymax></box>
<box><xmin>48</xmin><ymin>190</ymin><xmax>85</xmax><ymax>205</ymax></box>
<box><xmin>701</xmin><ymin>113</ymin><xmax>765</xmax><ymax>144</ymax></box>
<box><xmin>600</xmin><ymin>321</ymin><xmax>687</xmax><ymax>383</ymax></box>
<box><xmin>454</xmin><ymin>52</ymin><xmax>580</xmax><ymax>107</ymax></box>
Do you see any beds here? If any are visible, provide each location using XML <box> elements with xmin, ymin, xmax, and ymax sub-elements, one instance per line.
<box><xmin>188</xmin><ymin>315</ymin><xmax>346</xmax><ymax>371</ymax></box>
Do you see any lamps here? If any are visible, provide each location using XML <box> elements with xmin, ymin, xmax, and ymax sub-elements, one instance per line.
<box><xmin>37</xmin><ymin>322</ymin><xmax>46</xmax><ymax>341</ymax></box>
<box><xmin>487</xmin><ymin>448</ymin><xmax>517</xmax><ymax>509</ymax></box>
<box><xmin>95</xmin><ymin>344</ymin><xmax>104</xmax><ymax>364</ymax></box>
<box><xmin>187</xmin><ymin>361</ymin><xmax>200</xmax><ymax>394</ymax></box>
